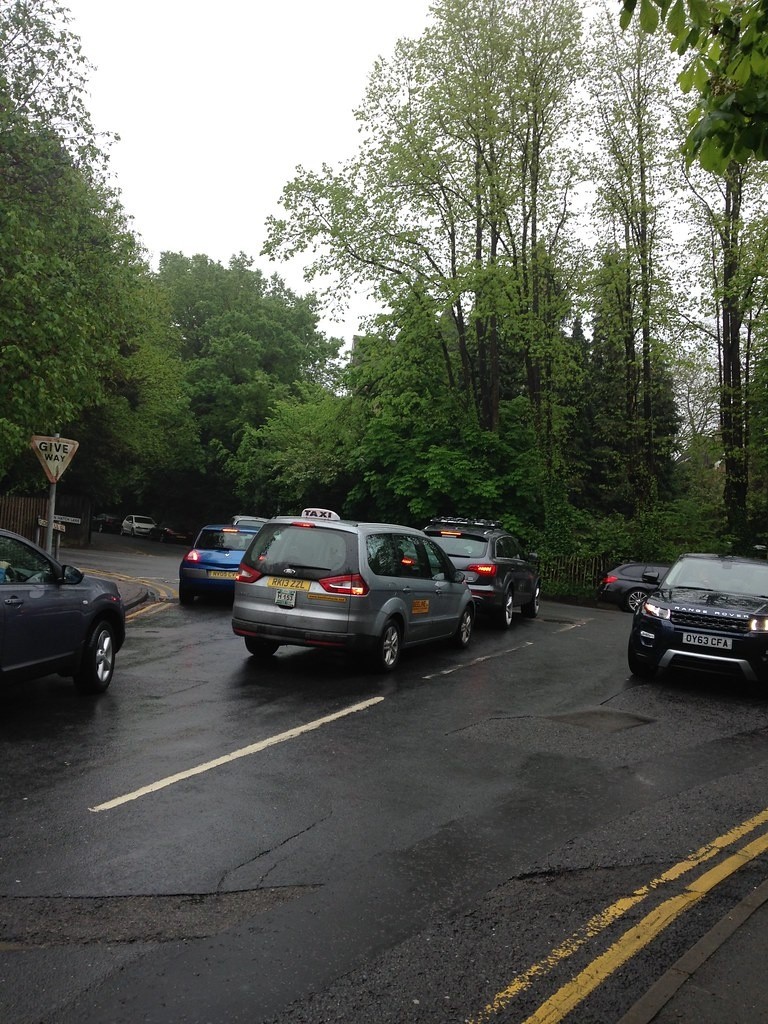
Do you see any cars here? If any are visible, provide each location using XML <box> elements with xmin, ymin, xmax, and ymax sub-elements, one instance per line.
<box><xmin>160</xmin><ymin>516</ymin><xmax>204</xmax><ymax>544</ymax></box>
<box><xmin>0</xmin><ymin>527</ymin><xmax>128</xmax><ymax>699</ymax></box>
<box><xmin>92</xmin><ymin>513</ymin><xmax>119</xmax><ymax>534</ymax></box>
<box><xmin>597</xmin><ymin>563</ymin><xmax>668</xmax><ymax>612</ymax></box>
<box><xmin>626</xmin><ymin>550</ymin><xmax>768</xmax><ymax>684</ymax></box>
<box><xmin>180</xmin><ymin>521</ymin><xmax>271</xmax><ymax>601</ymax></box>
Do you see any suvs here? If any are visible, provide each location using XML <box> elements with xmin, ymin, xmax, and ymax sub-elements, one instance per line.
<box><xmin>120</xmin><ymin>514</ymin><xmax>157</xmax><ymax>537</ymax></box>
<box><xmin>418</xmin><ymin>515</ymin><xmax>543</xmax><ymax>632</ymax></box>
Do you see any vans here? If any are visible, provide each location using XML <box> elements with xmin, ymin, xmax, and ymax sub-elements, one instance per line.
<box><xmin>229</xmin><ymin>507</ymin><xmax>478</xmax><ymax>670</ymax></box>
